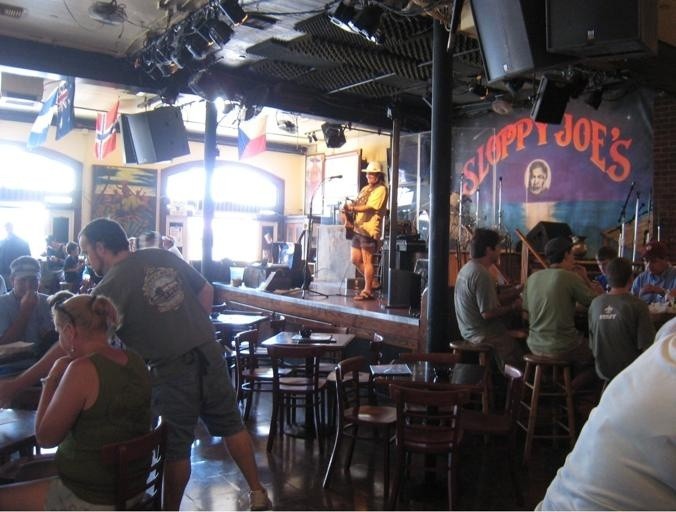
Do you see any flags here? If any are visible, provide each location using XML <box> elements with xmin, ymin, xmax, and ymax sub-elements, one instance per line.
<box><xmin>236</xmin><ymin>116</ymin><xmax>267</xmax><ymax>162</ymax></box>
<box><xmin>53</xmin><ymin>79</ymin><xmax>78</xmax><ymax>143</ymax></box>
<box><xmin>18</xmin><ymin>87</ymin><xmax>59</xmax><ymax>151</ymax></box>
<box><xmin>91</xmin><ymin>100</ymin><xmax>122</xmax><ymax>163</ymax></box>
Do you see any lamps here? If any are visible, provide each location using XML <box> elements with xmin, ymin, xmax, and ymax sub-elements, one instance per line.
<box><xmin>469</xmin><ymin>58</ymin><xmax>532</xmax><ymax>105</ymax></box>
<box><xmin>222</xmin><ymin>91</ymin><xmax>264</xmax><ymax>120</ymax></box>
<box><xmin>306</xmin><ymin>128</ymin><xmax>321</xmax><ymax>146</ymax></box>
<box><xmin>563</xmin><ymin>60</ymin><xmax>609</xmax><ymax>113</ymax></box>
<box><xmin>322</xmin><ymin>1</ymin><xmax>394</xmax><ymax>49</ymax></box>
<box><xmin>128</xmin><ymin>0</ymin><xmax>250</xmax><ymax>85</ymax></box>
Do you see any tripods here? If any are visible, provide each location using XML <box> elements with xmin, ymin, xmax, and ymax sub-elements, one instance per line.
<box><xmin>449</xmin><ymin>182</ymin><xmax>513</xmax><ymax>273</ymax></box>
<box><xmin>281</xmin><ymin>177</ymin><xmax>328</xmax><ymax>299</ymax></box>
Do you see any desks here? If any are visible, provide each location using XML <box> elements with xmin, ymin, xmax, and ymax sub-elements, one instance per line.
<box><xmin>511</xmin><ymin>289</ymin><xmax>675</xmax><ymax>346</ymax></box>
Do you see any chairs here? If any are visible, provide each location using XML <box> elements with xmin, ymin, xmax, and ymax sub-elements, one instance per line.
<box><xmin>1</xmin><ymin>252</ymin><xmax>167</xmax><ymax>510</ymax></box>
<box><xmin>205</xmin><ymin>303</ymin><xmax>495</xmax><ymax>511</ymax></box>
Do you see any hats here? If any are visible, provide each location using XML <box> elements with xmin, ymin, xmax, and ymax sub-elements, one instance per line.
<box><xmin>641</xmin><ymin>241</ymin><xmax>668</xmax><ymax>258</ymax></box>
<box><xmin>10</xmin><ymin>265</ymin><xmax>42</xmax><ymax>278</ymax></box>
<box><xmin>571</xmin><ymin>236</ymin><xmax>587</xmax><ymax>245</ymax></box>
<box><xmin>162</xmin><ymin>235</ymin><xmax>175</xmax><ymax>241</ymax></box>
<box><xmin>361</xmin><ymin>161</ymin><xmax>386</xmax><ymax>177</ymax></box>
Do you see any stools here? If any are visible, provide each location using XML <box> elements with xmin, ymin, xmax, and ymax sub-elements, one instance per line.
<box><xmin>516</xmin><ymin>348</ymin><xmax>584</xmax><ymax>468</ymax></box>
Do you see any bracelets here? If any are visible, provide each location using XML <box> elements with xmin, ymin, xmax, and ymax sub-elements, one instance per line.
<box><xmin>39</xmin><ymin>373</ymin><xmax>61</xmax><ymax>385</ymax></box>
<box><xmin>350</xmin><ymin>206</ymin><xmax>355</xmax><ymax>211</ymax></box>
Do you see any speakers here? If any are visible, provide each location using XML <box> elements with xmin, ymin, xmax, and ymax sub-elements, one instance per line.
<box><xmin>468</xmin><ymin>0</ymin><xmax>556</xmax><ymax>85</ymax></box>
<box><xmin>52</xmin><ymin>217</ymin><xmax>69</xmax><ymax>244</ymax></box>
<box><xmin>388</xmin><ymin>268</ymin><xmax>422</xmax><ymax>308</ymax></box>
<box><xmin>545</xmin><ymin>0</ymin><xmax>658</xmax><ymax>61</ymax></box>
<box><xmin>126</xmin><ymin>107</ymin><xmax>191</xmax><ymax>165</ymax></box>
<box><xmin>243</xmin><ymin>263</ymin><xmax>290</xmax><ymax>291</ymax></box>
<box><xmin>118</xmin><ymin>114</ymin><xmax>137</xmax><ymax>165</ymax></box>
<box><xmin>516</xmin><ymin>221</ymin><xmax>572</xmax><ymax>253</ymax></box>
<box><xmin>381</xmin><ymin>251</ymin><xmax>417</xmax><ymax>302</ymax></box>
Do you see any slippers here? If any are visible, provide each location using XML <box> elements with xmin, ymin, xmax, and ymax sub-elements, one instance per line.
<box><xmin>354</xmin><ymin>286</ymin><xmax>382</xmax><ymax>301</ymax></box>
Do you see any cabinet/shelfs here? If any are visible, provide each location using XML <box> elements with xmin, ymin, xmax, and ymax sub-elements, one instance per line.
<box><xmin>256</xmin><ymin>220</ymin><xmax>284</xmax><ymax>269</ymax></box>
<box><xmin>284</xmin><ymin>214</ymin><xmax>327</xmax><ymax>279</ymax></box>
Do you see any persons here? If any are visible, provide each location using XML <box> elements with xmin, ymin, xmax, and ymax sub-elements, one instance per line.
<box><xmin>527</xmin><ymin>161</ymin><xmax>548</xmax><ymax>197</ymax></box>
<box><xmin>532</xmin><ymin>314</ymin><xmax>676</xmax><ymax>511</ymax></box>
<box><xmin>0</xmin><ymin>218</ymin><xmax>273</xmax><ymax>511</ymax></box>
<box><xmin>523</xmin><ymin>237</ymin><xmax>656</xmax><ymax>389</ymax></box>
<box><xmin>457</xmin><ymin>227</ymin><xmax>527</xmax><ymax>384</ymax></box>
<box><xmin>0</xmin><ymin>220</ymin><xmax>185</xmax><ymax>410</ymax></box>
<box><xmin>630</xmin><ymin>238</ymin><xmax>674</xmax><ymax>304</ymax></box>
<box><xmin>262</xmin><ymin>232</ymin><xmax>273</xmax><ymax>260</ymax></box>
<box><xmin>340</xmin><ymin>160</ymin><xmax>388</xmax><ymax>302</ymax></box>
<box><xmin>32</xmin><ymin>294</ymin><xmax>154</xmax><ymax>511</ymax></box>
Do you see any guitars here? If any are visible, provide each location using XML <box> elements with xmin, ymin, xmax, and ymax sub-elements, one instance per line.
<box><xmin>342</xmin><ymin>198</ymin><xmax>355</xmax><ymax>240</ymax></box>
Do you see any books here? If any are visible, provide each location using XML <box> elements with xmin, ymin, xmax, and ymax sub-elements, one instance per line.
<box><xmin>292</xmin><ymin>331</ymin><xmax>337</xmax><ymax>343</ymax></box>
<box><xmin>369</xmin><ymin>362</ymin><xmax>412</xmax><ymax>376</ymax></box>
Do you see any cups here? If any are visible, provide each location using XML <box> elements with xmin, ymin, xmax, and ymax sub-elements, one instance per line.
<box><xmin>228</xmin><ymin>266</ymin><xmax>245</xmax><ymax>288</ymax></box>
<box><xmin>211</xmin><ymin>311</ymin><xmax>219</xmax><ymax>319</ymax></box>
<box><xmin>299</xmin><ymin>327</ymin><xmax>312</xmax><ymax>338</ymax></box>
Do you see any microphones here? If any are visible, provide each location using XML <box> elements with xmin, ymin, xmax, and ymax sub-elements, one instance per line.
<box><xmin>630</xmin><ymin>181</ymin><xmax>635</xmax><ymax>191</ymax></box>
<box><xmin>331</xmin><ymin>175</ymin><xmax>343</xmax><ymax>179</ymax></box>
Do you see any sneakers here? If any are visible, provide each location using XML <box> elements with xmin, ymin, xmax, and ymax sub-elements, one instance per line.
<box><xmin>249</xmin><ymin>487</ymin><xmax>272</xmax><ymax>511</ymax></box>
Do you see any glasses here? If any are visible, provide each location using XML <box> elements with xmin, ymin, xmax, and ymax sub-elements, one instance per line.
<box><xmin>52</xmin><ymin>298</ymin><xmax>75</xmax><ymax>328</ymax></box>
